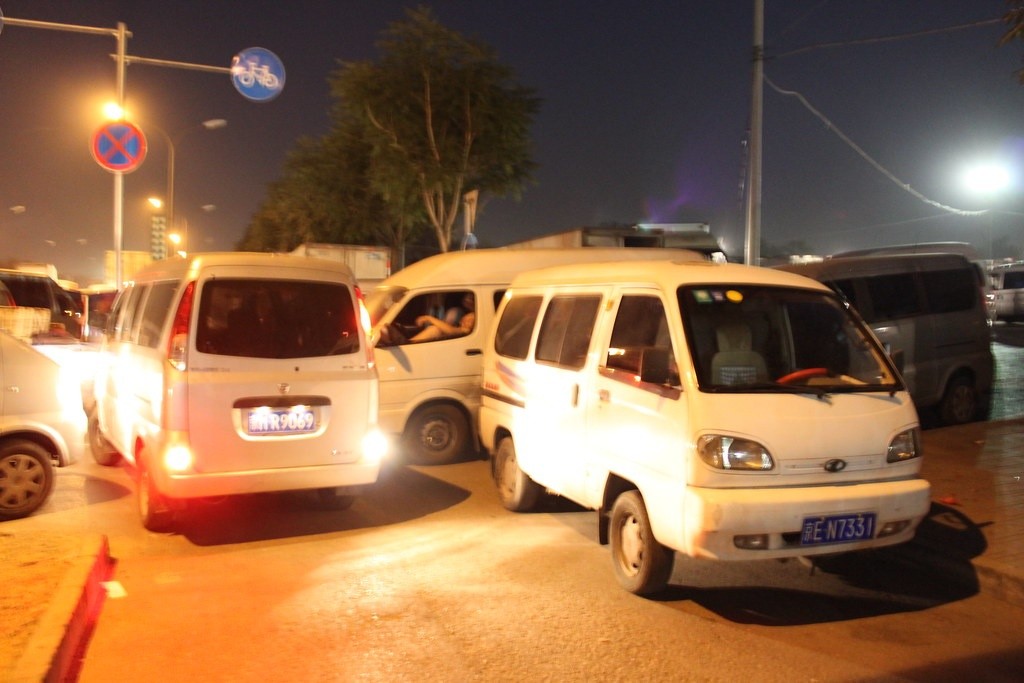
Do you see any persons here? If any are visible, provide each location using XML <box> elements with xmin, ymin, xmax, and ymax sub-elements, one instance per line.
<box><xmin>391</xmin><ymin>291</ymin><xmax>476</xmax><ymax>342</ymax></box>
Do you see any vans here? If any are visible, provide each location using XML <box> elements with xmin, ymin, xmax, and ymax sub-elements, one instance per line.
<box><xmin>81</xmin><ymin>251</ymin><xmax>384</xmax><ymax>530</ymax></box>
<box><xmin>979</xmin><ymin>262</ymin><xmax>1024</xmax><ymax>321</ymax></box>
<box><xmin>0</xmin><ymin>268</ymin><xmax>115</xmax><ymax>518</ymax></box>
<box><xmin>366</xmin><ymin>244</ymin><xmax>725</xmax><ymax>467</ymax></box>
<box><xmin>480</xmin><ymin>259</ymin><xmax>928</xmax><ymax>598</ymax></box>
<box><xmin>771</xmin><ymin>242</ymin><xmax>996</xmax><ymax>428</ymax></box>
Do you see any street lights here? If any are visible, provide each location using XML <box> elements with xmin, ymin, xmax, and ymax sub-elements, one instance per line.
<box><xmin>149</xmin><ymin>194</ymin><xmax>190</xmax><ymax>274</ymax></box>
<box><xmin>105</xmin><ymin>104</ymin><xmax>172</xmax><ymax>287</ymax></box>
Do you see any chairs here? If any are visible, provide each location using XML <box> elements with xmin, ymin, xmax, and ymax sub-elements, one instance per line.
<box><xmin>711</xmin><ymin>321</ymin><xmax>766</xmax><ymax>387</ymax></box>
